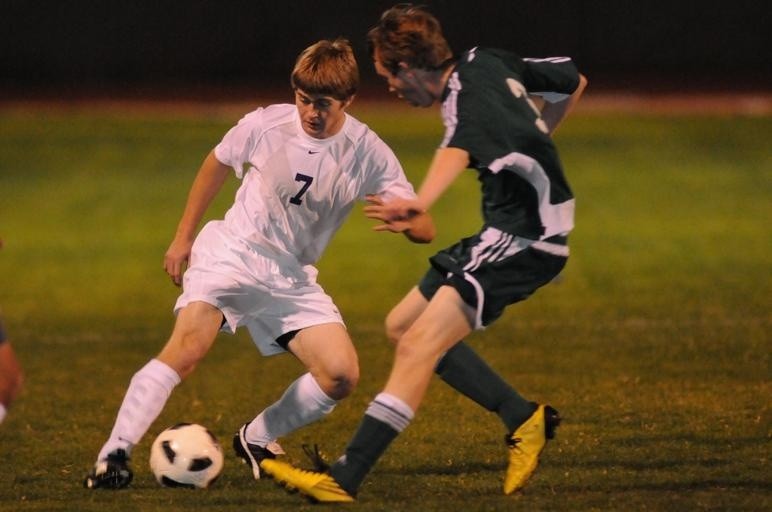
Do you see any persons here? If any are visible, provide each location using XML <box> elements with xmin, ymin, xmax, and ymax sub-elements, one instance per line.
<box><xmin>82</xmin><ymin>35</ymin><xmax>437</xmax><ymax>496</ymax></box>
<box><xmin>258</xmin><ymin>2</ymin><xmax>590</xmax><ymax>507</ymax></box>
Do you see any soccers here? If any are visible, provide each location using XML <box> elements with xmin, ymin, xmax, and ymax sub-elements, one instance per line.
<box><xmin>149</xmin><ymin>423</ymin><xmax>224</xmax><ymax>489</ymax></box>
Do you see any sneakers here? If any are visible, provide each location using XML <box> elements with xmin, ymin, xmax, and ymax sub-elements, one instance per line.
<box><xmin>504</xmin><ymin>399</ymin><xmax>561</xmax><ymax>494</ymax></box>
<box><xmin>84</xmin><ymin>448</ymin><xmax>132</xmax><ymax>489</ymax></box>
<box><xmin>232</xmin><ymin>422</ymin><xmax>285</xmax><ymax>480</ymax></box>
<box><xmin>259</xmin><ymin>456</ymin><xmax>358</xmax><ymax>503</ymax></box>
